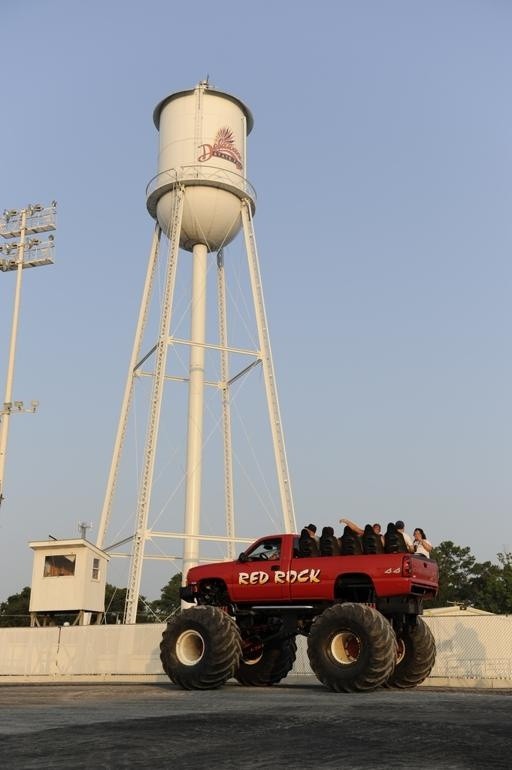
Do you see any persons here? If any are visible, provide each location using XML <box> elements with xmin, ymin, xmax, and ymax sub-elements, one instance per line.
<box><xmin>268</xmin><ymin>544</ymin><xmax>280</xmax><ymax>560</ymax></box>
<box><xmin>304</xmin><ymin>518</ymin><xmax>433</xmax><ymax>559</ymax></box>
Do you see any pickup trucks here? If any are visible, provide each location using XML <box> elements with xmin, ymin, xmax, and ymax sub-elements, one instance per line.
<box><xmin>160</xmin><ymin>534</ymin><xmax>439</xmax><ymax>693</ymax></box>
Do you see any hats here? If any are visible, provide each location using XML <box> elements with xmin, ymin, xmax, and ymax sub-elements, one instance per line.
<box><xmin>304</xmin><ymin>523</ymin><xmax>316</xmax><ymax>532</ymax></box>
